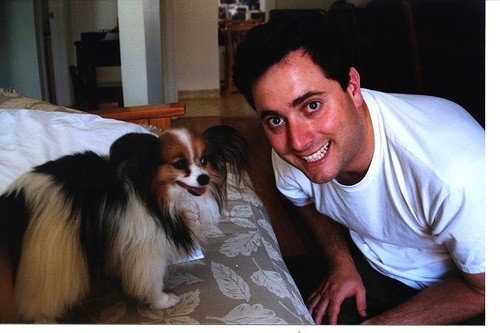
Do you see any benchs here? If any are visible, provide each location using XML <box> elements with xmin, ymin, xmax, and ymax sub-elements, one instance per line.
<box><xmin>0</xmin><ymin>100</ymin><xmax>316</xmax><ymax>325</ymax></box>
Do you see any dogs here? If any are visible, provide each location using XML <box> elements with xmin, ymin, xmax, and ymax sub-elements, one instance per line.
<box><xmin>0</xmin><ymin>124</ymin><xmax>251</xmax><ymax>325</ymax></box>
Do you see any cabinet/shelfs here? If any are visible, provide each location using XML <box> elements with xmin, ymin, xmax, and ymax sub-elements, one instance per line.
<box><xmin>219</xmin><ymin>0</ymin><xmax>268</xmax><ymax>43</ymax></box>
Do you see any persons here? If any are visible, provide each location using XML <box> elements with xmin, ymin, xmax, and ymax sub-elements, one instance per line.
<box><xmin>232</xmin><ymin>15</ymin><xmax>485</xmax><ymax>325</ymax></box>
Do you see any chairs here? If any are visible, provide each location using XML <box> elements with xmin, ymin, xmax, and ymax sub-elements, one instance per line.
<box><xmin>224</xmin><ymin>20</ymin><xmax>261</xmax><ymax>96</ymax></box>
